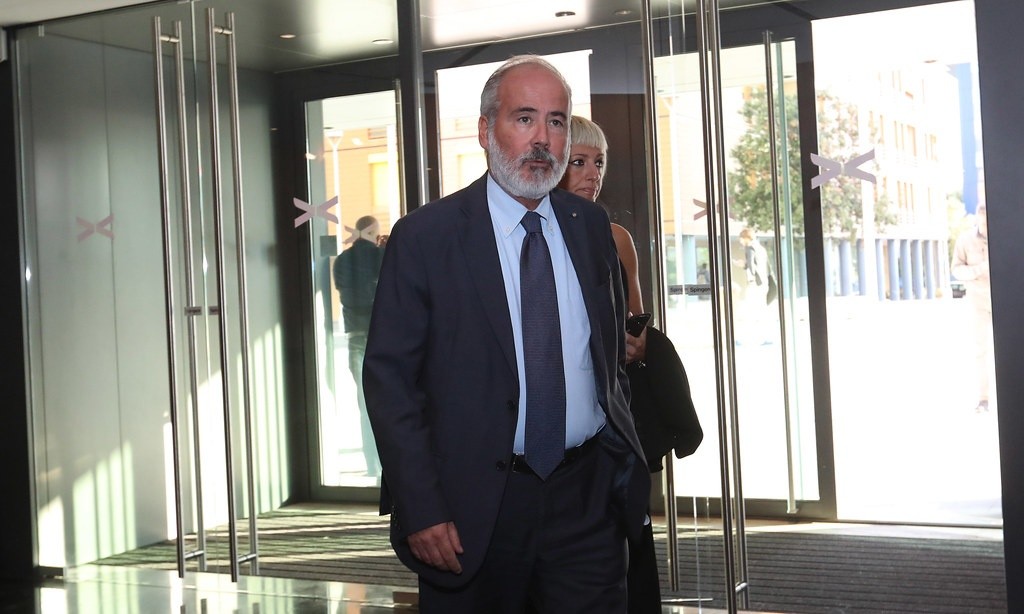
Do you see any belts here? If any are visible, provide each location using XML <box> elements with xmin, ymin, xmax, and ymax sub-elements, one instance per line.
<box><xmin>508</xmin><ymin>449</ymin><xmax>574</xmax><ymax>475</ymax></box>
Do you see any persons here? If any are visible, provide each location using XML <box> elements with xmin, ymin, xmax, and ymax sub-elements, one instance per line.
<box><xmin>332</xmin><ymin>214</ymin><xmax>390</xmax><ymax>478</ymax></box>
<box><xmin>360</xmin><ymin>54</ymin><xmax>704</xmax><ymax>614</ymax></box>
<box><xmin>732</xmin><ymin>229</ymin><xmax>777</xmax><ymax>350</ymax></box>
<box><xmin>696</xmin><ymin>262</ymin><xmax>711</xmax><ymax>301</ymax></box>
<box><xmin>950</xmin><ymin>203</ymin><xmax>999</xmax><ymax>415</ymax></box>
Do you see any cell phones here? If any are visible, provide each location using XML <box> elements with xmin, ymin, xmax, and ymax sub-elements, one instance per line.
<box><xmin>625</xmin><ymin>313</ymin><xmax>652</xmax><ymax>338</ymax></box>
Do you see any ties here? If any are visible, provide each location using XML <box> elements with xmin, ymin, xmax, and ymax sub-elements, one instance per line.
<box><xmin>518</xmin><ymin>209</ymin><xmax>567</xmax><ymax>485</ymax></box>
